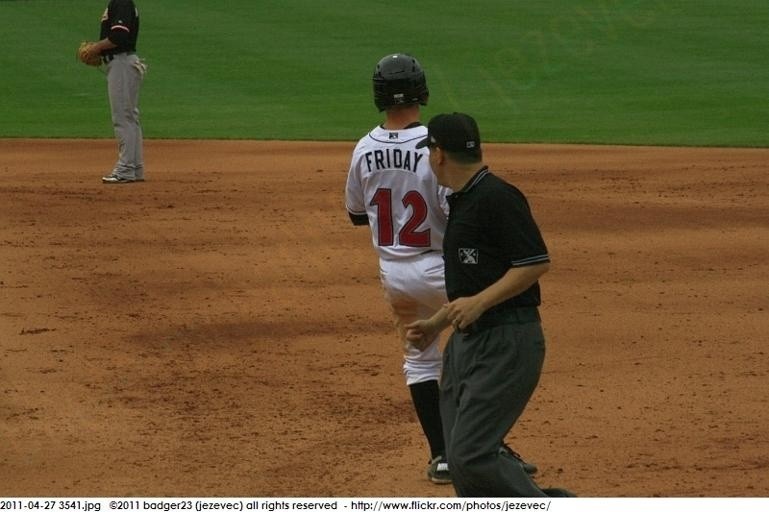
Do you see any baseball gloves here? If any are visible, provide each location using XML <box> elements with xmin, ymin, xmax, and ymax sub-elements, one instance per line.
<box><xmin>79</xmin><ymin>41</ymin><xmax>102</xmax><ymax>65</ymax></box>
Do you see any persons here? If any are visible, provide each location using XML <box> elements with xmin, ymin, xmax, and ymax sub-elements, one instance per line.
<box><xmin>79</xmin><ymin>0</ymin><xmax>149</xmax><ymax>186</ymax></box>
<box><xmin>397</xmin><ymin>111</ymin><xmax>574</xmax><ymax>497</ymax></box>
<box><xmin>343</xmin><ymin>53</ymin><xmax>455</xmax><ymax>485</ymax></box>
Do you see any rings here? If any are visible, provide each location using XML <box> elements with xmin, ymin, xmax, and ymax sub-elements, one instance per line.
<box><xmin>454</xmin><ymin>317</ymin><xmax>462</xmax><ymax>324</ymax></box>
